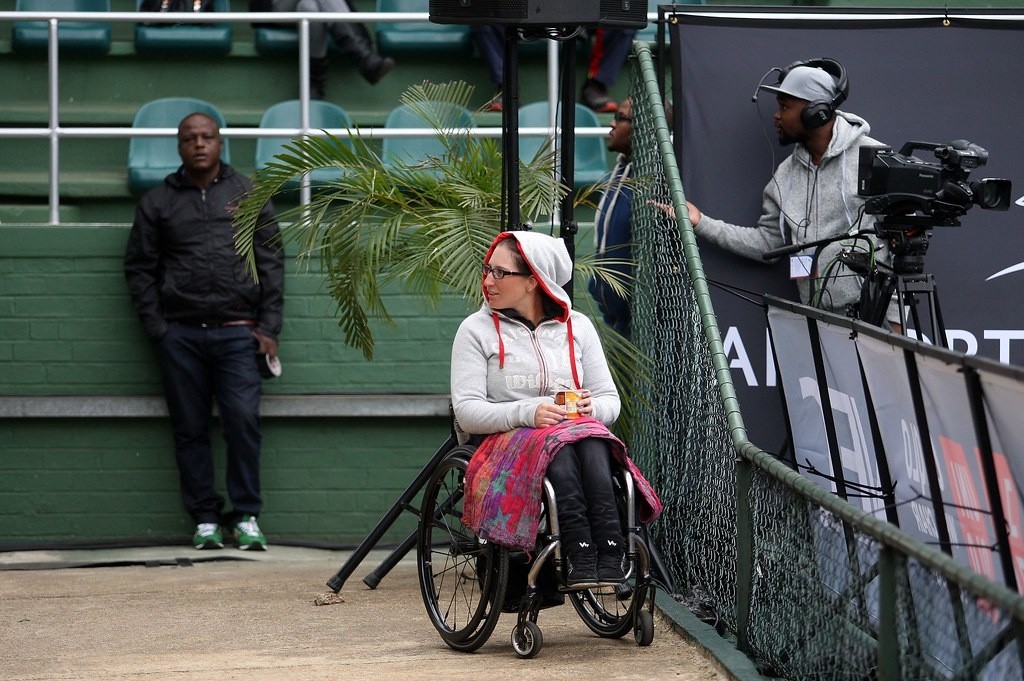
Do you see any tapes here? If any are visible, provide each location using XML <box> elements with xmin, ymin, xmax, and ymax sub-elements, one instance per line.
<box><xmin>256</xmin><ymin>352</ymin><xmax>282</xmax><ymax>379</ymax></box>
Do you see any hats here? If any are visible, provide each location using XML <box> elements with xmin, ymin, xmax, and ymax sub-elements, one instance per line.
<box><xmin>760</xmin><ymin>66</ymin><xmax>840</xmax><ymax>104</ymax></box>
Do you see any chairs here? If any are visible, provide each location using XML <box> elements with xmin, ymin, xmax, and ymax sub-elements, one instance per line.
<box><xmin>382</xmin><ymin>102</ymin><xmax>483</xmax><ymax>185</ymax></box>
<box><xmin>256</xmin><ymin>100</ymin><xmax>356</xmax><ymax>200</ymax></box>
<box><xmin>127</xmin><ymin>99</ymin><xmax>230</xmax><ymax>198</ymax></box>
<box><xmin>11</xmin><ymin>1</ymin><xmax>469</xmax><ymax>67</ymax></box>
<box><xmin>519</xmin><ymin>101</ymin><xmax>610</xmax><ymax>191</ymax></box>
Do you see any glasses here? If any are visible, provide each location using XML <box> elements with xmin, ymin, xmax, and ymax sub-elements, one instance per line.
<box><xmin>614</xmin><ymin>112</ymin><xmax>633</xmax><ymax>122</ymax></box>
<box><xmin>484</xmin><ymin>264</ymin><xmax>524</xmax><ymax>280</ymax></box>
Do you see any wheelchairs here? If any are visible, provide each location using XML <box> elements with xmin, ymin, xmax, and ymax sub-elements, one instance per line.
<box><xmin>325</xmin><ymin>398</ymin><xmax>657</xmax><ymax>658</ymax></box>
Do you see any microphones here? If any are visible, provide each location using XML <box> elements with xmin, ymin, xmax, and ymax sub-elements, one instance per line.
<box><xmin>751</xmin><ymin>67</ymin><xmax>783</xmax><ymax>103</ymax></box>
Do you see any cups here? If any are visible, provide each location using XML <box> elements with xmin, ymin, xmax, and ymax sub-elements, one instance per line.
<box><xmin>549</xmin><ymin>389</ymin><xmax>589</xmax><ymax>420</ymax></box>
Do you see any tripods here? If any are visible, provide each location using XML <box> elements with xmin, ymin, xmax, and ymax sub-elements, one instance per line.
<box><xmin>761</xmin><ymin>215</ymin><xmax>951</xmax><ymax>350</ymax></box>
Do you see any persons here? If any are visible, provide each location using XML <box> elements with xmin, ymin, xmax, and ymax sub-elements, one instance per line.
<box><xmin>124</xmin><ymin>112</ymin><xmax>285</xmax><ymax>551</ymax></box>
<box><xmin>646</xmin><ymin>63</ymin><xmax>913</xmax><ymax>334</ymax></box>
<box><xmin>450</xmin><ymin>230</ymin><xmax>626</xmax><ymax>589</ymax></box>
<box><xmin>471</xmin><ymin>23</ymin><xmax>634</xmax><ymax>112</ymax></box>
<box><xmin>585</xmin><ymin>93</ymin><xmax>673</xmax><ymax>372</ymax></box>
<box><xmin>248</xmin><ymin>0</ymin><xmax>394</xmax><ymax>101</ymax></box>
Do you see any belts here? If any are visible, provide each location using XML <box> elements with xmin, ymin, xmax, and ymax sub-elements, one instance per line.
<box><xmin>220</xmin><ymin>319</ymin><xmax>255</xmax><ymax>327</ymax></box>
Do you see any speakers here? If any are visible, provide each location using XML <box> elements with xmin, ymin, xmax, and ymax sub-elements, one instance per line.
<box><xmin>429</xmin><ymin>0</ymin><xmax>648</xmax><ymax>30</ymax></box>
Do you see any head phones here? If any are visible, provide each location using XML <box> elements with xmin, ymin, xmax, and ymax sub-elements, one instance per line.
<box><xmin>778</xmin><ymin>58</ymin><xmax>850</xmax><ymax>129</ymax></box>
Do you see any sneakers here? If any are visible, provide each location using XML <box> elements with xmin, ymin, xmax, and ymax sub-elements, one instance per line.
<box><xmin>231</xmin><ymin>513</ymin><xmax>269</xmax><ymax>551</ymax></box>
<box><xmin>597</xmin><ymin>539</ymin><xmax>626</xmax><ymax>581</ymax></box>
<box><xmin>490</xmin><ymin>91</ymin><xmax>503</xmax><ymax>112</ymax></box>
<box><xmin>192</xmin><ymin>523</ymin><xmax>225</xmax><ymax>550</ymax></box>
<box><xmin>581</xmin><ymin>80</ymin><xmax>617</xmax><ymax>114</ymax></box>
<box><xmin>566</xmin><ymin>540</ymin><xmax>598</xmax><ymax>583</ymax></box>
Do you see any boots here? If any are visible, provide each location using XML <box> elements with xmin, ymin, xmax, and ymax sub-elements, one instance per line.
<box><xmin>330</xmin><ymin>22</ymin><xmax>393</xmax><ymax>86</ymax></box>
<box><xmin>310</xmin><ymin>52</ymin><xmax>329</xmax><ymax>100</ymax></box>
